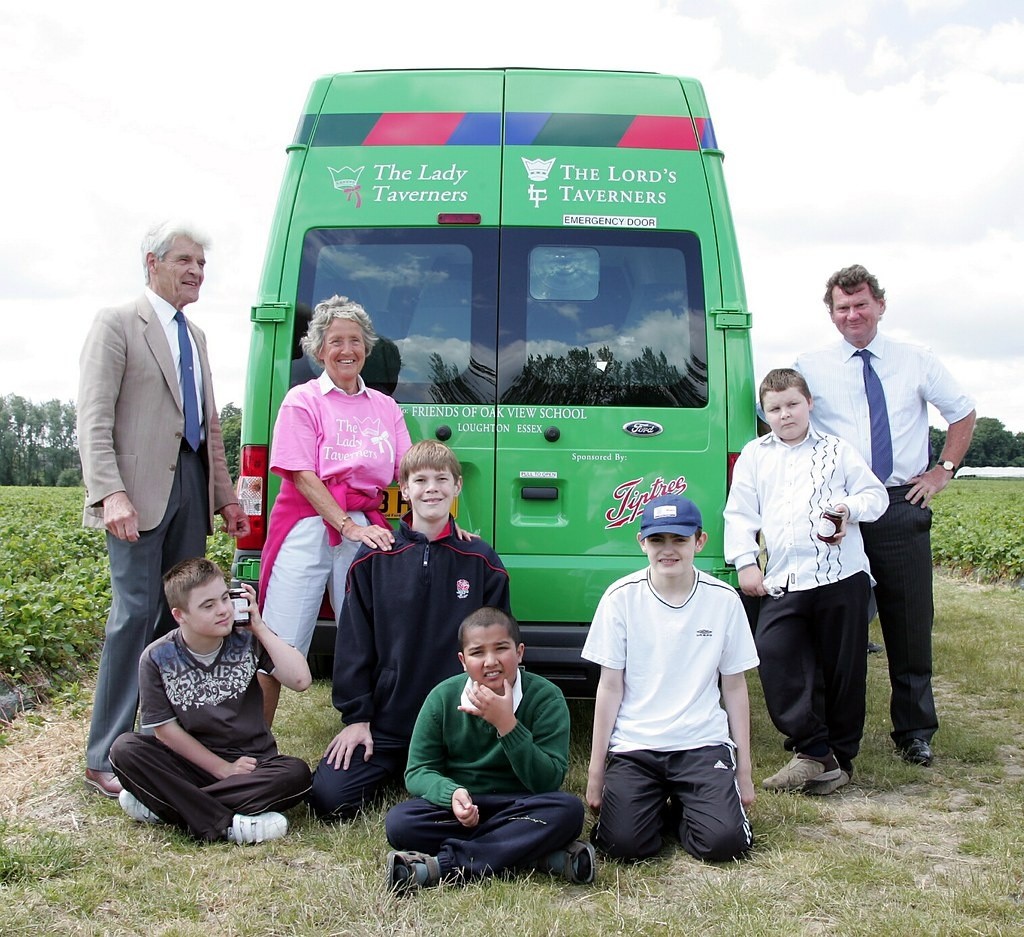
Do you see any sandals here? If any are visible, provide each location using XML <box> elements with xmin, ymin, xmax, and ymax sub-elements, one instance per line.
<box><xmin>385</xmin><ymin>850</ymin><xmax>437</xmax><ymax>896</ymax></box>
<box><xmin>541</xmin><ymin>840</ymin><xmax>596</xmax><ymax>884</ymax></box>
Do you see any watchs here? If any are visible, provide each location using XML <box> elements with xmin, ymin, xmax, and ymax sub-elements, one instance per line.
<box><xmin>937</xmin><ymin>459</ymin><xmax>955</xmax><ymax>479</ymax></box>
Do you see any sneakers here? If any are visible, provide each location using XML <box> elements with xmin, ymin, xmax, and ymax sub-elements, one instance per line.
<box><xmin>804</xmin><ymin>762</ymin><xmax>854</xmax><ymax>795</ymax></box>
<box><xmin>762</xmin><ymin>746</ymin><xmax>842</xmax><ymax>791</ymax></box>
<box><xmin>227</xmin><ymin>811</ymin><xmax>290</xmax><ymax>845</ymax></box>
<box><xmin>119</xmin><ymin>788</ymin><xmax>166</xmax><ymax>824</ymax></box>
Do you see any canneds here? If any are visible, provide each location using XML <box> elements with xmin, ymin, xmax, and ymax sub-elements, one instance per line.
<box><xmin>817</xmin><ymin>507</ymin><xmax>845</xmax><ymax>543</ymax></box>
<box><xmin>228</xmin><ymin>588</ymin><xmax>250</xmax><ymax>626</ymax></box>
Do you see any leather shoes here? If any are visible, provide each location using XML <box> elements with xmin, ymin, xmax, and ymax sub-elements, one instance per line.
<box><xmin>896</xmin><ymin>739</ymin><xmax>934</xmax><ymax>766</ymax></box>
<box><xmin>82</xmin><ymin>769</ymin><xmax>122</xmax><ymax>797</ymax></box>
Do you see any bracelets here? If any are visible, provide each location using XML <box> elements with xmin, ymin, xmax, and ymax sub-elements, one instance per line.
<box><xmin>339</xmin><ymin>515</ymin><xmax>352</xmax><ymax>536</ymax></box>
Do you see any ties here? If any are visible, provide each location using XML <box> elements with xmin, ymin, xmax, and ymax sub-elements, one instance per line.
<box><xmin>173</xmin><ymin>310</ymin><xmax>201</xmax><ymax>452</ymax></box>
<box><xmin>853</xmin><ymin>351</ymin><xmax>893</xmax><ymax>484</ymax></box>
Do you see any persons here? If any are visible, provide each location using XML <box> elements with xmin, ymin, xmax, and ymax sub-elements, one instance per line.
<box><xmin>722</xmin><ymin>368</ymin><xmax>890</xmax><ymax>797</ymax></box>
<box><xmin>107</xmin><ymin>554</ymin><xmax>313</xmax><ymax>846</ymax></box>
<box><xmin>256</xmin><ymin>292</ymin><xmax>481</xmax><ymax>732</ymax></box>
<box><xmin>755</xmin><ymin>266</ymin><xmax>976</xmax><ymax>766</ymax></box>
<box><xmin>580</xmin><ymin>493</ymin><xmax>760</xmax><ymax>865</ymax></box>
<box><xmin>384</xmin><ymin>608</ymin><xmax>595</xmax><ymax>896</ymax></box>
<box><xmin>74</xmin><ymin>216</ymin><xmax>251</xmax><ymax>797</ymax></box>
<box><xmin>310</xmin><ymin>440</ymin><xmax>514</xmax><ymax>824</ymax></box>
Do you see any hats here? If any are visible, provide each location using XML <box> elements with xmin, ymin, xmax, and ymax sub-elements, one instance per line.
<box><xmin>641</xmin><ymin>494</ymin><xmax>703</xmax><ymax>541</ymax></box>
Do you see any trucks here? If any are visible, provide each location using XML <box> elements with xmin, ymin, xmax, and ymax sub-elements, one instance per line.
<box><xmin>226</xmin><ymin>67</ymin><xmax>762</xmax><ymax>701</ymax></box>
